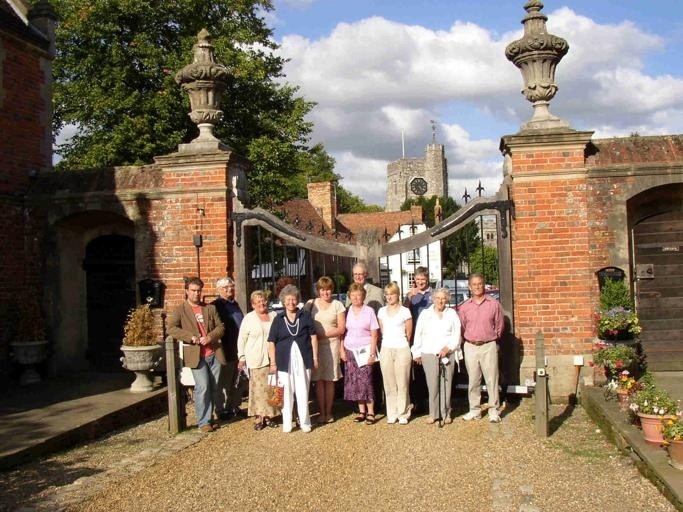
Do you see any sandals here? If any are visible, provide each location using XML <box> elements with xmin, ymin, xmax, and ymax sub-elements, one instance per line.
<box><xmin>254</xmin><ymin>419</ymin><xmax>262</xmax><ymax>431</ymax></box>
<box><xmin>366</xmin><ymin>414</ymin><xmax>376</xmax><ymax>426</ymax></box>
<box><xmin>264</xmin><ymin>419</ymin><xmax>277</xmax><ymax>430</ymax></box>
<box><xmin>354</xmin><ymin>411</ymin><xmax>366</xmax><ymax>422</ymax></box>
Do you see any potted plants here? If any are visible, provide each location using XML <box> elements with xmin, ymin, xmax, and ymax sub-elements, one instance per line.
<box><xmin>119</xmin><ymin>301</ymin><xmax>164</xmax><ymax>393</ymax></box>
<box><xmin>8</xmin><ymin>291</ymin><xmax>49</xmax><ymax>388</ymax></box>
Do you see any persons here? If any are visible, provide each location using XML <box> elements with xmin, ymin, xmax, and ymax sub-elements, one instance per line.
<box><xmin>345</xmin><ymin>263</ymin><xmax>385</xmax><ymax>315</ymax></box>
<box><xmin>411</xmin><ymin>286</ymin><xmax>461</xmax><ymax>424</ymax></box>
<box><xmin>210</xmin><ymin>278</ymin><xmax>245</xmax><ymax>410</ymax></box>
<box><xmin>236</xmin><ymin>275</ymin><xmax>346</xmax><ymax>433</ymax></box>
<box><xmin>403</xmin><ymin>266</ymin><xmax>436</xmax><ymax>409</ymax></box>
<box><xmin>458</xmin><ymin>274</ymin><xmax>505</xmax><ymax>422</ymax></box>
<box><xmin>377</xmin><ymin>281</ymin><xmax>412</xmax><ymax>424</ymax></box>
<box><xmin>168</xmin><ymin>277</ymin><xmax>226</xmax><ymax>433</ymax></box>
<box><xmin>339</xmin><ymin>283</ymin><xmax>380</xmax><ymax>425</ymax></box>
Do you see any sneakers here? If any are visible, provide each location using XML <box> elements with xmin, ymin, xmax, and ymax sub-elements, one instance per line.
<box><xmin>462</xmin><ymin>408</ymin><xmax>482</xmax><ymax>423</ymax></box>
<box><xmin>488</xmin><ymin>408</ymin><xmax>499</xmax><ymax>424</ymax></box>
<box><xmin>301</xmin><ymin>425</ymin><xmax>312</xmax><ymax>433</ymax></box>
<box><xmin>387</xmin><ymin>417</ymin><xmax>397</xmax><ymax>424</ymax></box>
<box><xmin>398</xmin><ymin>416</ymin><xmax>408</xmax><ymax>425</ymax></box>
<box><xmin>282</xmin><ymin>426</ymin><xmax>293</xmax><ymax>433</ymax></box>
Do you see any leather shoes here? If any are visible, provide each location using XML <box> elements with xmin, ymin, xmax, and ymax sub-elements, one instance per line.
<box><xmin>199</xmin><ymin>423</ymin><xmax>214</xmax><ymax>433</ymax></box>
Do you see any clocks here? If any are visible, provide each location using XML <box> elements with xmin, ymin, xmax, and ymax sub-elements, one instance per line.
<box><xmin>410</xmin><ymin>177</ymin><xmax>428</xmax><ymax>195</ymax></box>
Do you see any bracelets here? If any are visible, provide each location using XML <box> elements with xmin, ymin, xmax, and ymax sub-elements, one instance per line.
<box><xmin>370</xmin><ymin>355</ymin><xmax>375</xmax><ymax>358</ymax></box>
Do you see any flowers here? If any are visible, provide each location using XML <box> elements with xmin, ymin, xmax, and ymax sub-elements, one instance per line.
<box><xmin>587</xmin><ymin>303</ymin><xmax>682</xmax><ymax>471</ymax></box>
